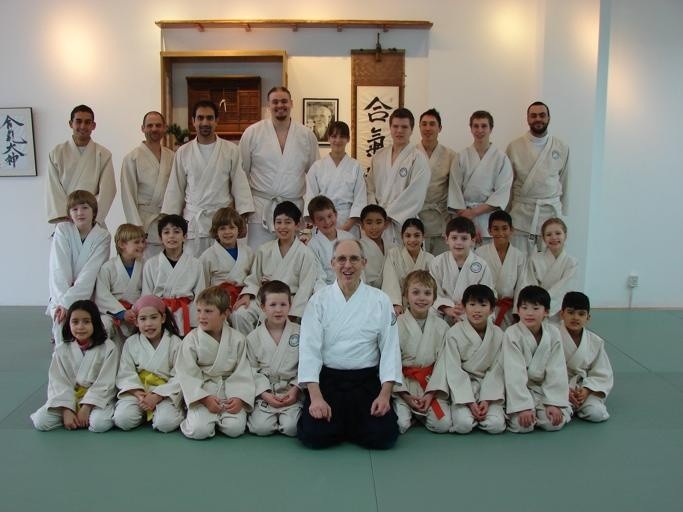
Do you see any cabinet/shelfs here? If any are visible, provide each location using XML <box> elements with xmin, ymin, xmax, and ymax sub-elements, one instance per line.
<box><xmin>184</xmin><ymin>75</ymin><xmax>263</xmax><ymax>141</ymax></box>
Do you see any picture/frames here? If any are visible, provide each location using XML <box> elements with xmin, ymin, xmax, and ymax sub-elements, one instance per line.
<box><xmin>303</xmin><ymin>98</ymin><xmax>339</xmax><ymax>146</ymax></box>
<box><xmin>0</xmin><ymin>106</ymin><xmax>37</xmax><ymax>178</ymax></box>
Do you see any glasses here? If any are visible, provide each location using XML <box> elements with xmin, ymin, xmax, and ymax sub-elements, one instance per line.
<box><xmin>333</xmin><ymin>255</ymin><xmax>362</xmax><ymax>265</ymax></box>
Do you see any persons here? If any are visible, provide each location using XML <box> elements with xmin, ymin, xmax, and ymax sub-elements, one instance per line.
<box><xmin>292</xmin><ymin>237</ymin><xmax>405</xmax><ymax>450</ymax></box>
<box><xmin>28</xmin><ymin>190</ymin><xmax>613</xmax><ymax>439</ymax></box>
<box><xmin>44</xmin><ymin>86</ymin><xmax>570</xmax><ymax>261</ymax></box>
